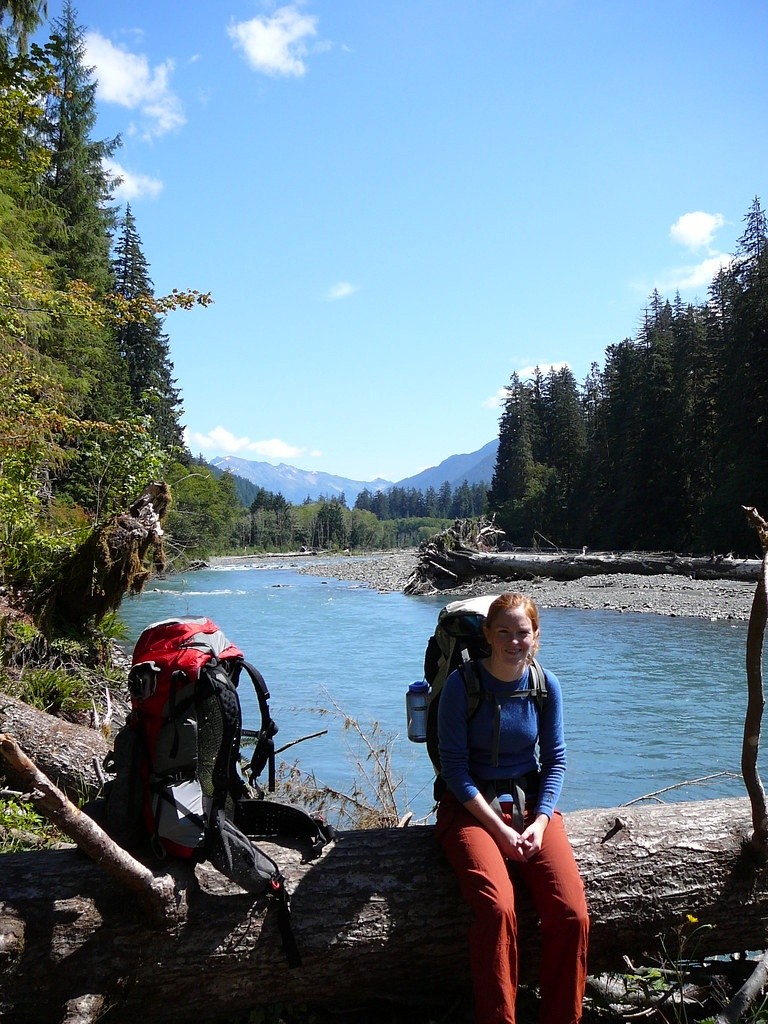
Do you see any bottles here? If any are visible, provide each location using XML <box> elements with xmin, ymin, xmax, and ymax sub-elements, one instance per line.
<box><xmin>406</xmin><ymin>676</ymin><xmax>432</xmax><ymax>743</ymax></box>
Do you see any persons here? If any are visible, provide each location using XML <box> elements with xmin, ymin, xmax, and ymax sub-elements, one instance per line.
<box><xmin>436</xmin><ymin>594</ymin><xmax>590</xmax><ymax>1024</ymax></box>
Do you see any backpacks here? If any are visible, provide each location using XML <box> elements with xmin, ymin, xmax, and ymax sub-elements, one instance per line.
<box><xmin>103</xmin><ymin>614</ymin><xmax>278</xmax><ymax>865</ymax></box>
<box><xmin>425</xmin><ymin>594</ymin><xmax>549</xmax><ymax>802</ymax></box>
